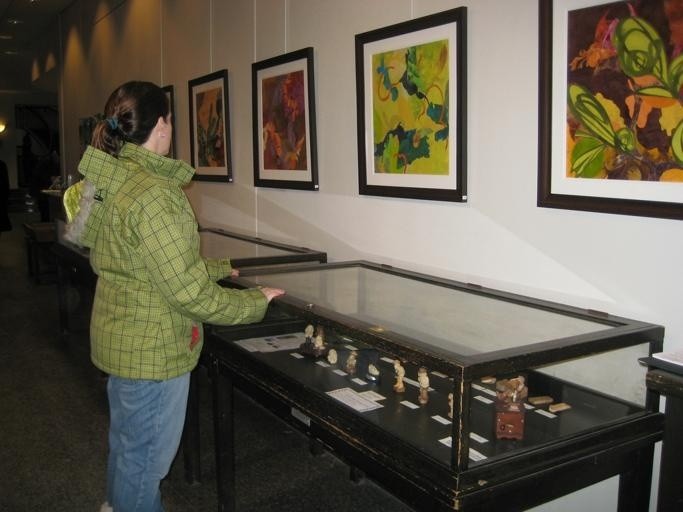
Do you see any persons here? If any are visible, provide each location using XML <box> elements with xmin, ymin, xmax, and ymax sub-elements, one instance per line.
<box><xmin>81</xmin><ymin>82</ymin><xmax>286</xmax><ymax>511</ymax></box>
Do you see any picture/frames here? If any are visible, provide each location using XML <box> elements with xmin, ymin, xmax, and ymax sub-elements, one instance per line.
<box><xmin>188</xmin><ymin>69</ymin><xmax>232</xmax><ymax>183</ymax></box>
<box><xmin>536</xmin><ymin>1</ymin><xmax>681</xmax><ymax>221</ymax></box>
<box><xmin>160</xmin><ymin>84</ymin><xmax>176</xmax><ymax>161</ymax></box>
<box><xmin>355</xmin><ymin>5</ymin><xmax>468</xmax><ymax>203</ymax></box>
<box><xmin>250</xmin><ymin>47</ymin><xmax>319</xmax><ymax>192</ymax></box>
